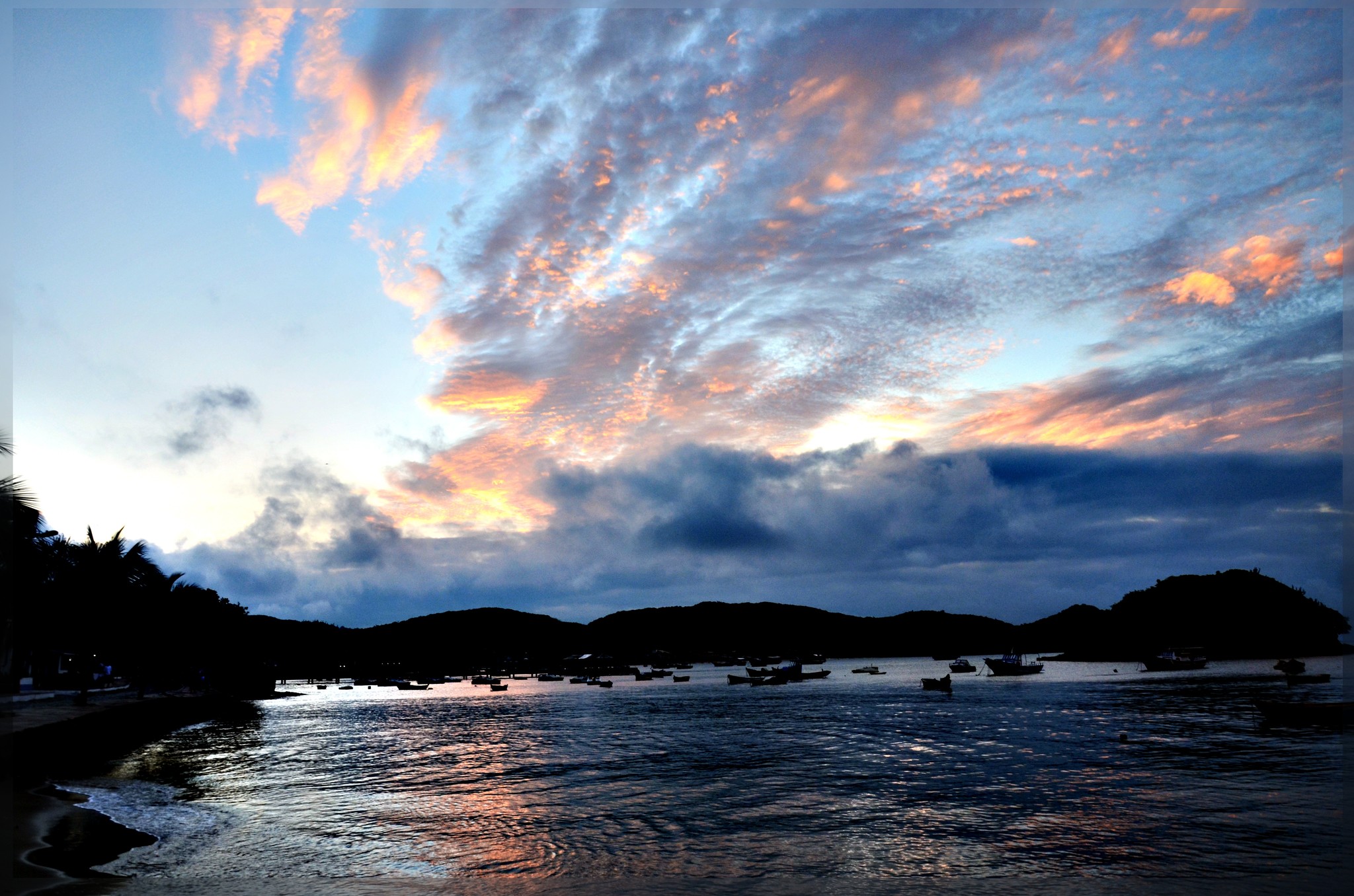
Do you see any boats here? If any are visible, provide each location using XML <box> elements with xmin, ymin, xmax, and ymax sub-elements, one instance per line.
<box><xmin>921</xmin><ymin>674</ymin><xmax>952</xmax><ymax>690</ymax></box>
<box><xmin>471</xmin><ymin>674</ymin><xmax>501</xmax><ymax>684</ymax></box>
<box><xmin>538</xmin><ymin>674</ymin><xmax>564</xmax><ymax>681</ymax></box>
<box><xmin>490</xmin><ymin>684</ymin><xmax>508</xmax><ymax>691</ymax></box>
<box><xmin>394</xmin><ymin>681</ymin><xmax>430</xmax><ymax>690</ymax></box>
<box><xmin>851</xmin><ymin>664</ymin><xmax>879</xmax><ymax>673</ymax></box>
<box><xmin>1273</xmin><ymin>657</ymin><xmax>1305</xmax><ymax>672</ymax></box>
<box><xmin>569</xmin><ymin>675</ymin><xmax>588</xmax><ymax>683</ymax></box>
<box><xmin>711</xmin><ymin>654</ymin><xmax>831</xmax><ymax>687</ymax></box>
<box><xmin>982</xmin><ymin>652</ymin><xmax>1044</xmax><ymax>676</ymax></box>
<box><xmin>949</xmin><ymin>659</ymin><xmax>976</xmax><ymax>673</ymax></box>
<box><xmin>1139</xmin><ymin>647</ymin><xmax>1208</xmax><ymax>671</ymax></box>
<box><xmin>869</xmin><ymin>672</ymin><xmax>887</xmax><ymax>675</ymax></box>
<box><xmin>444</xmin><ymin>676</ymin><xmax>462</xmax><ymax>682</ymax></box>
<box><xmin>600</xmin><ymin>681</ymin><xmax>613</xmax><ymax>688</ymax></box>
<box><xmin>634</xmin><ymin>662</ymin><xmax>694</xmax><ymax>682</ymax></box>
<box><xmin>1248</xmin><ymin>686</ymin><xmax>1354</xmax><ymax>727</ymax></box>
<box><xmin>317</xmin><ymin>685</ymin><xmax>327</xmax><ymax>689</ymax></box>
<box><xmin>416</xmin><ymin>677</ymin><xmax>447</xmax><ymax>684</ymax></box>
<box><xmin>587</xmin><ymin>676</ymin><xmax>601</xmax><ymax>685</ymax></box>
